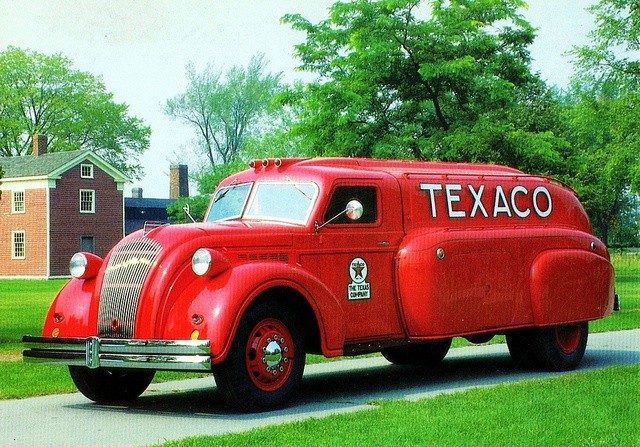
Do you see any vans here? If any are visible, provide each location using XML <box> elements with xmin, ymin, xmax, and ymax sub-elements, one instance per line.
<box><xmin>23</xmin><ymin>156</ymin><xmax>620</xmax><ymax>412</ymax></box>
